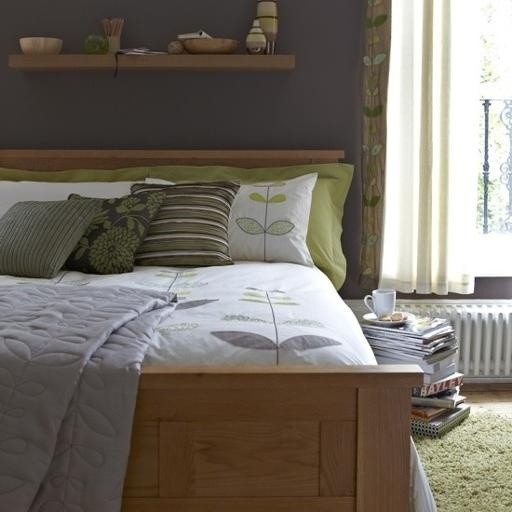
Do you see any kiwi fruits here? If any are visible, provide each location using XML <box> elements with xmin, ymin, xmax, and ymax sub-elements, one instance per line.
<box><xmin>168</xmin><ymin>42</ymin><xmax>184</xmax><ymax>54</ymax></box>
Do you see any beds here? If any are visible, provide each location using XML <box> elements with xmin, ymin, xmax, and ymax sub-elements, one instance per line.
<box><xmin>1</xmin><ymin>149</ymin><xmax>424</xmax><ymax>510</ymax></box>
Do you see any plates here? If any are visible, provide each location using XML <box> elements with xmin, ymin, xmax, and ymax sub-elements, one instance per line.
<box><xmin>362</xmin><ymin>311</ymin><xmax>416</xmax><ymax>327</ymax></box>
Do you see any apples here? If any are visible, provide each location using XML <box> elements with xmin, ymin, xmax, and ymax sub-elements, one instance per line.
<box><xmin>84</xmin><ymin>33</ymin><xmax>107</xmax><ymax>54</ymax></box>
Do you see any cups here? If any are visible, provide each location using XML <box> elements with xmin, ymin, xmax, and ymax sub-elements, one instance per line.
<box><xmin>364</xmin><ymin>289</ymin><xmax>397</xmax><ymax>319</ymax></box>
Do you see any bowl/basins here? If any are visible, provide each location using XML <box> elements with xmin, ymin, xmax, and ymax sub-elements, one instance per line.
<box><xmin>182</xmin><ymin>38</ymin><xmax>241</xmax><ymax>54</ymax></box>
<box><xmin>19</xmin><ymin>37</ymin><xmax>63</xmax><ymax>55</ymax></box>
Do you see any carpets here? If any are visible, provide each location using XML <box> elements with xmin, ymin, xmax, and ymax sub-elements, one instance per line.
<box><xmin>412</xmin><ymin>403</ymin><xmax>511</xmax><ymax>512</ymax></box>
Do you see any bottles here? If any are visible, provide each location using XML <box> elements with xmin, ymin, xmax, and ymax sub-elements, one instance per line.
<box><xmin>245</xmin><ymin>1</ymin><xmax>279</xmax><ymax>54</ymax></box>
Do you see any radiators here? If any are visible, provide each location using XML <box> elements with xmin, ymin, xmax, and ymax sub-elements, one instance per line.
<box><xmin>391</xmin><ymin>297</ymin><xmax>512</xmax><ymax>377</ymax></box>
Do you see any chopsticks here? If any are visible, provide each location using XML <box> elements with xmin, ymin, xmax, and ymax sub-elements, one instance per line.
<box><xmin>102</xmin><ymin>16</ymin><xmax>125</xmax><ymax>36</ymax></box>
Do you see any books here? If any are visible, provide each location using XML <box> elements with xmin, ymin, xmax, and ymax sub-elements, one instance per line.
<box><xmin>361</xmin><ymin>317</ymin><xmax>467</xmax><ymax>421</ymax></box>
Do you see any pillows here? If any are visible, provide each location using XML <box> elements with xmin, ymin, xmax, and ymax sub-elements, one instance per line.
<box><xmin>3</xmin><ymin>198</ymin><xmax>104</xmax><ymax>279</ymax></box>
<box><xmin>227</xmin><ymin>171</ymin><xmax>319</xmax><ymax>269</ymax></box>
<box><xmin>128</xmin><ymin>178</ymin><xmax>241</xmax><ymax>269</ymax></box>
<box><xmin>1</xmin><ymin>166</ymin><xmax>355</xmax><ymax>294</ymax></box>
<box><xmin>60</xmin><ymin>189</ymin><xmax>169</xmax><ymax>276</ymax></box>
